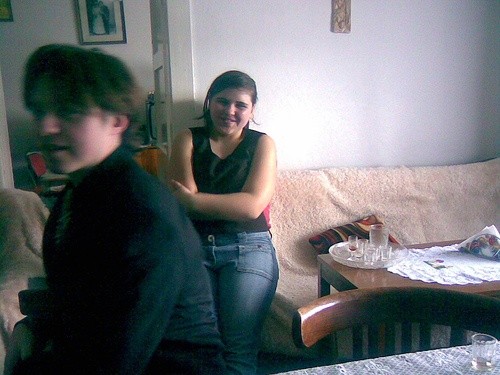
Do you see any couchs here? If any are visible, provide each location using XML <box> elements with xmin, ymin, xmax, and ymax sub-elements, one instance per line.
<box><xmin>259</xmin><ymin>157</ymin><xmax>500</xmax><ymax>358</ymax></box>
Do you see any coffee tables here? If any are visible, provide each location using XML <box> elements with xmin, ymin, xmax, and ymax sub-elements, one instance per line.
<box><xmin>318</xmin><ymin>240</ymin><xmax>500</xmax><ymax>358</ymax></box>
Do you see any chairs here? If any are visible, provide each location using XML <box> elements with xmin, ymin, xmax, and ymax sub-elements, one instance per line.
<box><xmin>25</xmin><ymin>151</ymin><xmax>73</xmax><ymax>199</ymax></box>
<box><xmin>291</xmin><ymin>285</ymin><xmax>500</xmax><ymax>364</ymax></box>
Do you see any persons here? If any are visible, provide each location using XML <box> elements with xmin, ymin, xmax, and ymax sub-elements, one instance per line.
<box><xmin>92</xmin><ymin>1</ymin><xmax>111</xmax><ymax>35</ymax></box>
<box><xmin>0</xmin><ymin>44</ymin><xmax>228</xmax><ymax>375</ymax></box>
<box><xmin>165</xmin><ymin>70</ymin><xmax>279</xmax><ymax>375</ymax></box>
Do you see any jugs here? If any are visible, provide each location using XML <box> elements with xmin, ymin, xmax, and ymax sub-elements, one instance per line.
<box><xmin>369</xmin><ymin>224</ymin><xmax>389</xmax><ymax>246</ymax></box>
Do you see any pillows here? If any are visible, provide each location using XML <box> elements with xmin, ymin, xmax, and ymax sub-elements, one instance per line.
<box><xmin>308</xmin><ymin>215</ymin><xmax>401</xmax><ymax>254</ymax></box>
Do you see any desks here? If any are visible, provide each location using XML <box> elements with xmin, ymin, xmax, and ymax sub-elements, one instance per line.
<box><xmin>274</xmin><ymin>342</ymin><xmax>500</xmax><ymax>375</ymax></box>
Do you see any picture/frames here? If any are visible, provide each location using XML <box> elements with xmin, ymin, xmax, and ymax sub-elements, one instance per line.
<box><xmin>0</xmin><ymin>0</ymin><xmax>14</xmax><ymax>23</ymax></box>
<box><xmin>74</xmin><ymin>0</ymin><xmax>127</xmax><ymax>46</ymax></box>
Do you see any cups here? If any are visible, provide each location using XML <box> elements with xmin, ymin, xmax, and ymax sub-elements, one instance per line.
<box><xmin>470</xmin><ymin>333</ymin><xmax>497</xmax><ymax>371</ymax></box>
<box><xmin>348</xmin><ymin>235</ymin><xmax>392</xmax><ymax>261</ymax></box>
